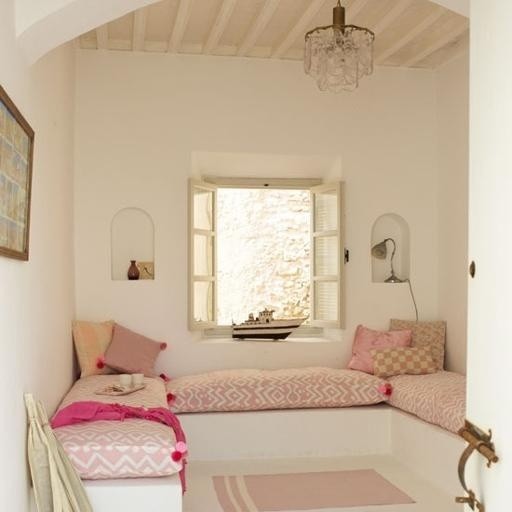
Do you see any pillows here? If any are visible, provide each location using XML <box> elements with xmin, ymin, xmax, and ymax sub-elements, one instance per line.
<box><xmin>97</xmin><ymin>323</ymin><xmax>170</xmax><ymax>377</ymax></box>
<box><xmin>72</xmin><ymin>319</ymin><xmax>114</xmax><ymax>379</ymax></box>
<box><xmin>388</xmin><ymin>317</ymin><xmax>447</xmax><ymax>372</ymax></box>
<box><xmin>370</xmin><ymin>345</ymin><xmax>438</xmax><ymax>375</ymax></box>
<box><xmin>347</xmin><ymin>325</ymin><xmax>412</xmax><ymax>373</ymax></box>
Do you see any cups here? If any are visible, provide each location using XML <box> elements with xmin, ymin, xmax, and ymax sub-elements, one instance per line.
<box><xmin>119</xmin><ymin>373</ymin><xmax>132</xmax><ymax>388</ymax></box>
<box><xmin>132</xmin><ymin>373</ymin><xmax>144</xmax><ymax>387</ymax></box>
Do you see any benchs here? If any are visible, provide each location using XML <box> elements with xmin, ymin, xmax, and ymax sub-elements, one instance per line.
<box><xmin>167</xmin><ymin>364</ymin><xmax>389</xmax><ymax>480</ymax></box>
<box><xmin>47</xmin><ymin>370</ymin><xmax>183</xmax><ymax>512</ymax></box>
<box><xmin>385</xmin><ymin>354</ymin><xmax>466</xmax><ymax>512</ymax></box>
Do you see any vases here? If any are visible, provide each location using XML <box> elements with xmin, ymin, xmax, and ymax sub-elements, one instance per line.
<box><xmin>128</xmin><ymin>259</ymin><xmax>140</xmax><ymax>280</ymax></box>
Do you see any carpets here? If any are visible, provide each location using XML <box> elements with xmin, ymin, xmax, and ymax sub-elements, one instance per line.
<box><xmin>210</xmin><ymin>467</ymin><xmax>416</xmax><ymax>512</ymax></box>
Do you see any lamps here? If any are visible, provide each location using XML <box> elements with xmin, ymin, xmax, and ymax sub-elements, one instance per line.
<box><xmin>300</xmin><ymin>5</ymin><xmax>375</xmax><ymax>96</ymax></box>
<box><xmin>370</xmin><ymin>237</ymin><xmax>403</xmax><ymax>283</ymax></box>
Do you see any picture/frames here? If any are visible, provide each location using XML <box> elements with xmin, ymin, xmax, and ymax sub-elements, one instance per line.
<box><xmin>0</xmin><ymin>85</ymin><xmax>35</xmax><ymax>264</ymax></box>
